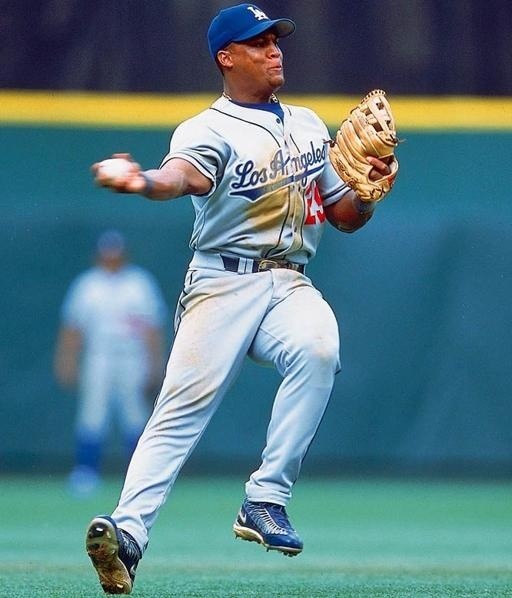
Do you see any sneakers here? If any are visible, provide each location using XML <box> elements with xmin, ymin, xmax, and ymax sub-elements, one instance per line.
<box><xmin>231</xmin><ymin>497</ymin><xmax>303</xmax><ymax>556</ymax></box>
<box><xmin>85</xmin><ymin>515</ymin><xmax>144</xmax><ymax>594</ymax></box>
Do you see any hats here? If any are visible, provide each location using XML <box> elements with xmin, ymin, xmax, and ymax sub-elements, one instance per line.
<box><xmin>206</xmin><ymin>3</ymin><xmax>297</xmax><ymax>61</ymax></box>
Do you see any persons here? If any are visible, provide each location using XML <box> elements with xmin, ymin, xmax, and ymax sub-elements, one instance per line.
<box><xmin>85</xmin><ymin>3</ymin><xmax>398</xmax><ymax>594</ymax></box>
<box><xmin>54</xmin><ymin>229</ymin><xmax>168</xmax><ymax>495</ymax></box>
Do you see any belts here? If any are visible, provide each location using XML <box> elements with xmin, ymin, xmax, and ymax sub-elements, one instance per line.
<box><xmin>220</xmin><ymin>254</ymin><xmax>305</xmax><ymax>274</ymax></box>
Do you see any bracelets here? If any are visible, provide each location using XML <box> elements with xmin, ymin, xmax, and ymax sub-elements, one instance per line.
<box><xmin>139</xmin><ymin>174</ymin><xmax>153</xmax><ymax>196</ymax></box>
<box><xmin>352</xmin><ymin>192</ymin><xmax>376</xmax><ymax>214</ymax></box>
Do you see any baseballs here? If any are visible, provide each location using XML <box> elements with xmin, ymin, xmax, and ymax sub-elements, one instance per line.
<box><xmin>99</xmin><ymin>158</ymin><xmax>134</xmax><ymax>181</ymax></box>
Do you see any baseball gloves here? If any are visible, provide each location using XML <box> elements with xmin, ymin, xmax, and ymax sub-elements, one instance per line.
<box><xmin>323</xmin><ymin>89</ymin><xmax>400</xmax><ymax>203</ymax></box>
